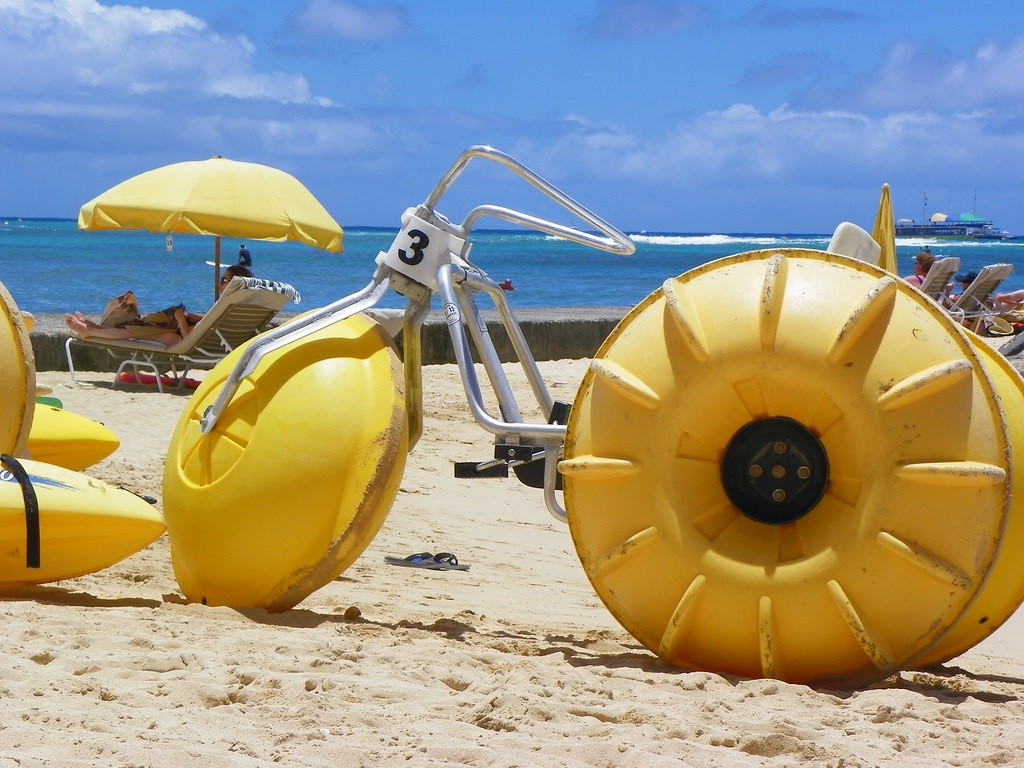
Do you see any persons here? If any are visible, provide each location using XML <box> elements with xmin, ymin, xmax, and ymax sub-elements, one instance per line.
<box><xmin>65</xmin><ymin>266</ymin><xmax>255</xmax><ymax>345</ymax></box>
<box><xmin>238</xmin><ymin>244</ymin><xmax>252</xmax><ymax>266</ymax></box>
<box><xmin>942</xmin><ymin>271</ymin><xmax>978</xmax><ymax>310</ymax></box>
<box><xmin>902</xmin><ymin>252</ymin><xmax>936</xmax><ymax>288</ymax></box>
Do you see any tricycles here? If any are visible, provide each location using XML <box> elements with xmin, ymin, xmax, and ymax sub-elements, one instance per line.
<box><xmin>160</xmin><ymin>143</ymin><xmax>1024</xmax><ymax>687</ymax></box>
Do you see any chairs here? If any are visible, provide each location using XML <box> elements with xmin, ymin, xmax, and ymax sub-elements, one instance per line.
<box><xmin>918</xmin><ymin>256</ymin><xmax>965</xmax><ymax>326</ymax></box>
<box><xmin>951</xmin><ymin>263</ymin><xmax>1015</xmax><ymax>333</ymax></box>
<box><xmin>65</xmin><ymin>275</ymin><xmax>301</xmax><ymax>394</ymax></box>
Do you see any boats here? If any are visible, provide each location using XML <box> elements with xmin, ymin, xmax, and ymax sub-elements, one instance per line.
<box><xmin>891</xmin><ymin>213</ymin><xmax>1009</xmax><ymax>239</ymax></box>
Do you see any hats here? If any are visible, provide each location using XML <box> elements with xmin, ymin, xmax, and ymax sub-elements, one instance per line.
<box><xmin>955</xmin><ymin>272</ymin><xmax>978</xmax><ymax>283</ymax></box>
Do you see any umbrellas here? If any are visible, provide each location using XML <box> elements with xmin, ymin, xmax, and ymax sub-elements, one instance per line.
<box><xmin>870</xmin><ymin>182</ymin><xmax>898</xmax><ymax>275</ymax></box>
<box><xmin>78</xmin><ymin>155</ymin><xmax>343</xmax><ymax>302</ymax></box>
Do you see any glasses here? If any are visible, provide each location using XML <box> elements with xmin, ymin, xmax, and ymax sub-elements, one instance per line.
<box><xmin>221</xmin><ymin>278</ymin><xmax>231</xmax><ymax>285</ymax></box>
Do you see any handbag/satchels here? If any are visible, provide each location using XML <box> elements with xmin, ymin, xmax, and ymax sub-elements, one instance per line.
<box><xmin>99</xmin><ymin>291</ymin><xmax>140</xmax><ymax>327</ymax></box>
